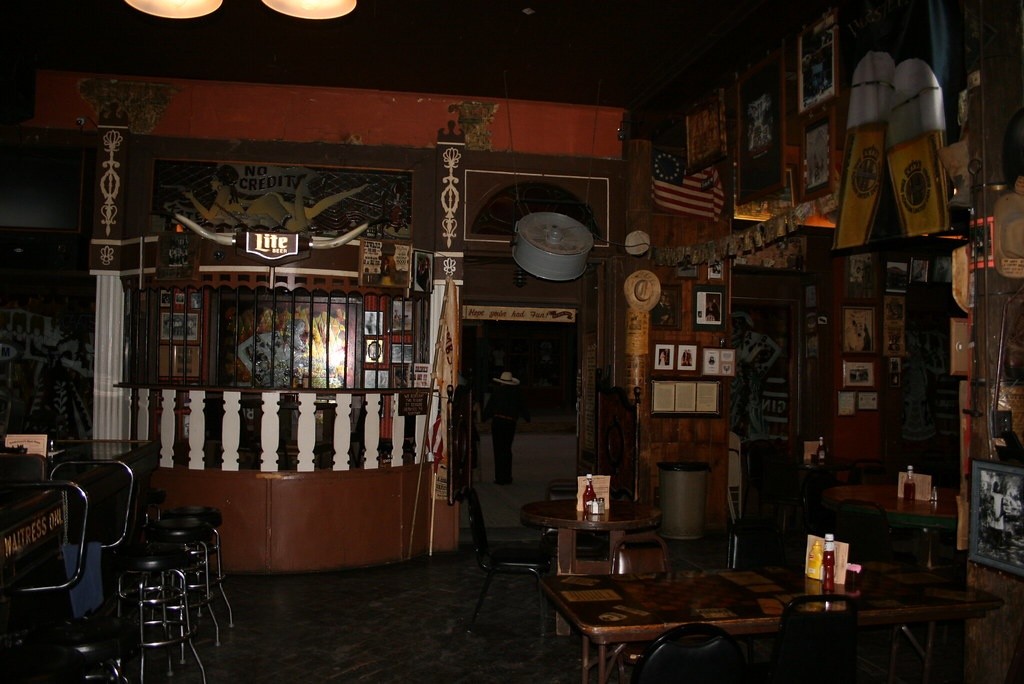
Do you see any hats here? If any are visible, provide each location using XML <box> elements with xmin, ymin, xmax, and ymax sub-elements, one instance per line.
<box><xmin>492</xmin><ymin>371</ymin><xmax>521</xmax><ymax>386</ymax></box>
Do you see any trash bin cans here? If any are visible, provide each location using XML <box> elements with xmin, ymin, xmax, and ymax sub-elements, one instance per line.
<box><xmin>657</xmin><ymin>460</ymin><xmax>709</xmax><ymax>540</ymax></box>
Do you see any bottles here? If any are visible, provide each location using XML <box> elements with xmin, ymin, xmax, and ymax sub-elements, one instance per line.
<box><xmin>592</xmin><ymin>498</ymin><xmax>598</xmax><ymax>515</ymax></box>
<box><xmin>822</xmin><ymin>533</ymin><xmax>835</xmax><ymax>595</ymax></box>
<box><xmin>816</xmin><ymin>437</ymin><xmax>826</xmax><ymax>463</ymax></box>
<box><xmin>583</xmin><ymin>474</ymin><xmax>596</xmax><ymax>514</ymax></box>
<box><xmin>598</xmin><ymin>498</ymin><xmax>605</xmax><ymax>514</ymax></box>
<box><xmin>904</xmin><ymin>466</ymin><xmax>915</xmax><ymax>501</ymax></box>
<box><xmin>931</xmin><ymin>487</ymin><xmax>938</xmax><ymax>500</ymax></box>
<box><xmin>807</xmin><ymin>540</ymin><xmax>823</xmax><ymax>580</ymax></box>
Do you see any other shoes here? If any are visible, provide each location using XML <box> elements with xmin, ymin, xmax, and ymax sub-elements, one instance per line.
<box><xmin>494</xmin><ymin>479</ymin><xmax>513</xmax><ymax>485</ymax></box>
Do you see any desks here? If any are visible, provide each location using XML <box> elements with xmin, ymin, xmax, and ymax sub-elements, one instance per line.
<box><xmin>520</xmin><ymin>501</ymin><xmax>660</xmax><ymax>573</ymax></box>
<box><xmin>823</xmin><ymin>481</ymin><xmax>959</xmax><ymax>557</ymax></box>
<box><xmin>537</xmin><ymin>559</ymin><xmax>1004</xmax><ymax>684</ymax></box>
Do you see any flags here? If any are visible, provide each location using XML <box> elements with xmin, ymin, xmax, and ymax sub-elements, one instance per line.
<box><xmin>649</xmin><ymin>151</ymin><xmax>725</xmax><ymax>225</ymax></box>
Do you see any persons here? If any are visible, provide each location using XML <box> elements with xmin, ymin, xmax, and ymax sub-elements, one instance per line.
<box><xmin>478</xmin><ymin>371</ymin><xmax>520</xmax><ymax>485</ymax></box>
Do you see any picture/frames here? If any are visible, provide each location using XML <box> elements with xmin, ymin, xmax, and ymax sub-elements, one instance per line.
<box><xmin>647</xmin><ymin>340</ymin><xmax>701</xmax><ymax>378</ymax></box>
<box><xmin>157</xmin><ymin>289</ymin><xmax>202</xmax><ymax>381</ymax></box>
<box><xmin>684</xmin><ymin>85</ymin><xmax>729</xmax><ymax>175</ymax></box>
<box><xmin>799</xmin><ymin>11</ymin><xmax>838</xmax><ymax>115</ymax></box>
<box><xmin>798</xmin><ymin>115</ymin><xmax>839</xmax><ymax>204</ymax></box>
<box><xmin>700</xmin><ymin>346</ymin><xmax>737</xmax><ymax>379</ymax></box>
<box><xmin>734</xmin><ymin>38</ymin><xmax>786</xmax><ymax>206</ymax></box>
<box><xmin>884</xmin><ymin>261</ymin><xmax>908</xmax><ymax>294</ymax></box>
<box><xmin>357</xmin><ymin>239</ymin><xmax>435</xmax><ymax>391</ymax></box>
<box><xmin>847</xmin><ymin>253</ymin><xmax>880</xmax><ymax>302</ymax></box>
<box><xmin>693</xmin><ymin>283</ymin><xmax>728</xmax><ymax>334</ymax></box>
<box><xmin>968</xmin><ymin>460</ymin><xmax>1024</xmax><ymax>577</ymax></box>
<box><xmin>646</xmin><ymin>282</ymin><xmax>684</xmax><ymax>332</ymax></box>
<box><xmin>840</xmin><ymin>303</ymin><xmax>883</xmax><ymax>418</ymax></box>
<box><xmin>910</xmin><ymin>256</ymin><xmax>928</xmax><ymax>285</ymax></box>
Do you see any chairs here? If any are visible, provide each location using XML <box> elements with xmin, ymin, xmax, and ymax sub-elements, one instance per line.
<box><xmin>728</xmin><ymin>431</ymin><xmax>839</xmax><ymax>572</ymax></box>
<box><xmin>631</xmin><ymin>624</ymin><xmax>745</xmax><ymax>684</ymax></box>
<box><xmin>769</xmin><ymin>595</ymin><xmax>858</xmax><ymax>684</ymax></box>
<box><xmin>614</xmin><ymin>534</ymin><xmax>672</xmax><ymax>573</ymax></box>
<box><xmin>469</xmin><ymin>487</ymin><xmax>546</xmax><ymax>637</ymax></box>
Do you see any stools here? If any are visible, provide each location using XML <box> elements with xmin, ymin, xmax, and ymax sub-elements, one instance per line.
<box><xmin>0</xmin><ymin>502</ymin><xmax>233</xmax><ymax>683</ymax></box>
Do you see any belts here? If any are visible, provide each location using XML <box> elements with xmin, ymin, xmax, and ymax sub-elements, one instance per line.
<box><xmin>494</xmin><ymin>414</ymin><xmax>513</xmax><ymax>420</ymax></box>
